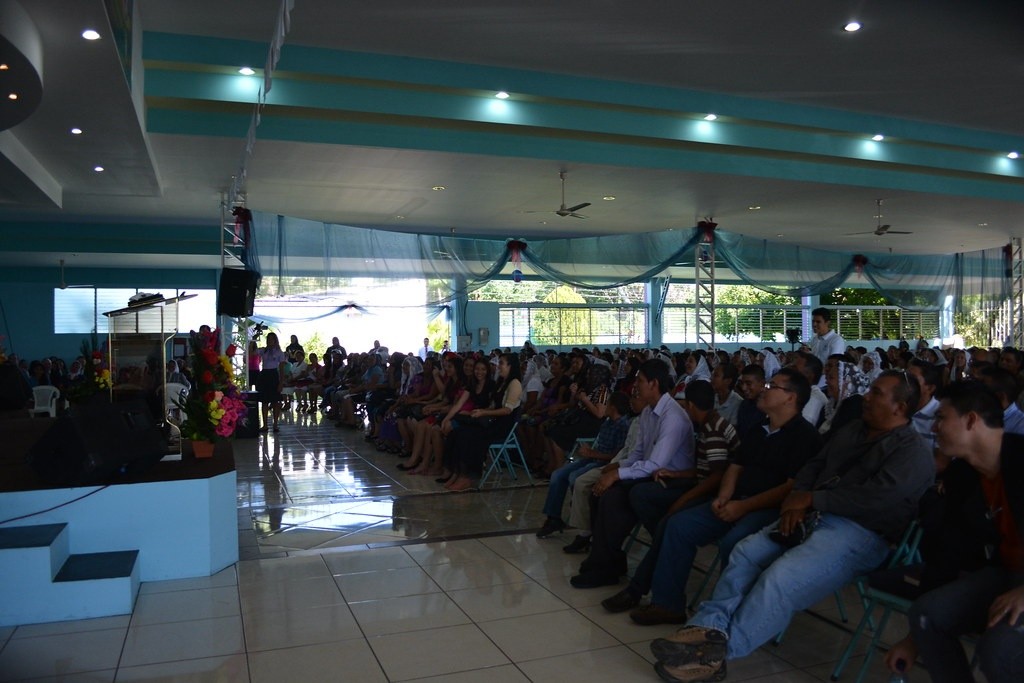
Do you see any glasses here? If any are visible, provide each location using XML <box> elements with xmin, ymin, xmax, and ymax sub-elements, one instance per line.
<box><xmin>763</xmin><ymin>382</ymin><xmax>797</xmax><ymax>394</ymax></box>
<box><xmin>887</xmin><ymin>365</ymin><xmax>912</xmax><ymax>397</ymax></box>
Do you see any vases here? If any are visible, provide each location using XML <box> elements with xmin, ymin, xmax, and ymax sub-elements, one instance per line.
<box><xmin>192</xmin><ymin>441</ymin><xmax>214</xmax><ymax>457</ymax></box>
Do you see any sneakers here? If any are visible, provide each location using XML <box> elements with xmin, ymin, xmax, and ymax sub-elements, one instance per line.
<box><xmin>630</xmin><ymin>603</ymin><xmax>689</xmax><ymax>626</ymax></box>
<box><xmin>570</xmin><ymin>555</ymin><xmax>620</xmax><ymax>588</ymax></box>
<box><xmin>536</xmin><ymin>515</ymin><xmax>566</xmax><ymax>539</ymax></box>
<box><xmin>652</xmin><ymin>658</ymin><xmax>728</xmax><ymax>683</ymax></box>
<box><xmin>599</xmin><ymin>585</ymin><xmax>640</xmax><ymax>611</ymax></box>
<box><xmin>649</xmin><ymin>621</ymin><xmax>728</xmax><ymax>668</ymax></box>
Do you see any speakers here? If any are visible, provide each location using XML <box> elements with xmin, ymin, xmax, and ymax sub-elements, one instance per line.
<box><xmin>218</xmin><ymin>267</ymin><xmax>260</xmax><ymax>318</ymax></box>
<box><xmin>32</xmin><ymin>403</ymin><xmax>165</xmax><ymax>484</ymax></box>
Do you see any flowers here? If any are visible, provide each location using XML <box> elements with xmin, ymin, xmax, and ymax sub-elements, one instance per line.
<box><xmin>78</xmin><ymin>329</ymin><xmax>112</xmax><ymax>392</ymax></box>
<box><xmin>166</xmin><ymin>326</ymin><xmax>250</xmax><ymax>443</ymax></box>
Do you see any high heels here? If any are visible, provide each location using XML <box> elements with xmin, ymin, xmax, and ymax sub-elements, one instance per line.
<box><xmin>562</xmin><ymin>533</ymin><xmax>593</xmax><ymax>555</ymax></box>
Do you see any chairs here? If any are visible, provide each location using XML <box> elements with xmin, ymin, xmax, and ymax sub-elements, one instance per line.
<box><xmin>568</xmin><ymin>436</ymin><xmax>598</xmax><ymax>461</ymax></box>
<box><xmin>769</xmin><ymin>573</ymin><xmax>875</xmax><ymax>648</ymax></box>
<box><xmin>624</xmin><ymin>523</ymin><xmax>652</xmax><ymax>566</ymax></box>
<box><xmin>29</xmin><ymin>385</ymin><xmax>60</xmax><ymax>419</ymax></box>
<box><xmin>686</xmin><ymin>542</ymin><xmax>722</xmax><ymax>611</ymax></box>
<box><xmin>591</xmin><ymin>417</ymin><xmax>611</xmax><ymax>452</ymax></box>
<box><xmin>356</xmin><ymin>402</ymin><xmax>370</xmax><ymax>430</ymax></box>
<box><xmin>832</xmin><ymin>517</ymin><xmax>924</xmax><ymax>681</ymax></box>
<box><xmin>477</xmin><ymin>421</ymin><xmax>535</xmax><ymax>489</ymax></box>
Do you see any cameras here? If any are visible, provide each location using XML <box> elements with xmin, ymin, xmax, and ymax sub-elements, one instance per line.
<box><xmin>787</xmin><ymin>330</ymin><xmax>802</xmax><ymax>344</ymax></box>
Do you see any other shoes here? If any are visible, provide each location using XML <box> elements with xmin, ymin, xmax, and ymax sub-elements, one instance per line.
<box><xmin>444</xmin><ymin>476</ymin><xmax>460</xmax><ymax>489</ymax></box>
<box><xmin>434</xmin><ymin>473</ymin><xmax>453</xmax><ymax>483</ymax></box>
<box><xmin>260</xmin><ymin>404</ymin><xmax>429</xmax><ymax>476</ymax></box>
<box><xmin>449</xmin><ymin>478</ymin><xmax>474</xmax><ymax>491</ymax></box>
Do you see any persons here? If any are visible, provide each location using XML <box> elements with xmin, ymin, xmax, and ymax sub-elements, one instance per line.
<box><xmin>246</xmin><ymin>331</ymin><xmax>1024</xmax><ymax>563</ymax></box>
<box><xmin>569</xmin><ymin>359</ymin><xmax>699</xmax><ymax>590</ymax></box>
<box><xmin>599</xmin><ymin>377</ymin><xmax>742</xmax><ymax>614</ymax></box>
<box><xmin>650</xmin><ymin>366</ymin><xmax>936</xmax><ymax>683</ymax></box>
<box><xmin>628</xmin><ymin>365</ymin><xmax>827</xmax><ymax>627</ymax></box>
<box><xmin>0</xmin><ymin>351</ymin><xmax>195</xmax><ymax>416</ymax></box>
<box><xmin>883</xmin><ymin>377</ymin><xmax>1024</xmax><ymax>682</ymax></box>
<box><xmin>801</xmin><ymin>307</ymin><xmax>848</xmax><ymax>363</ymax></box>
